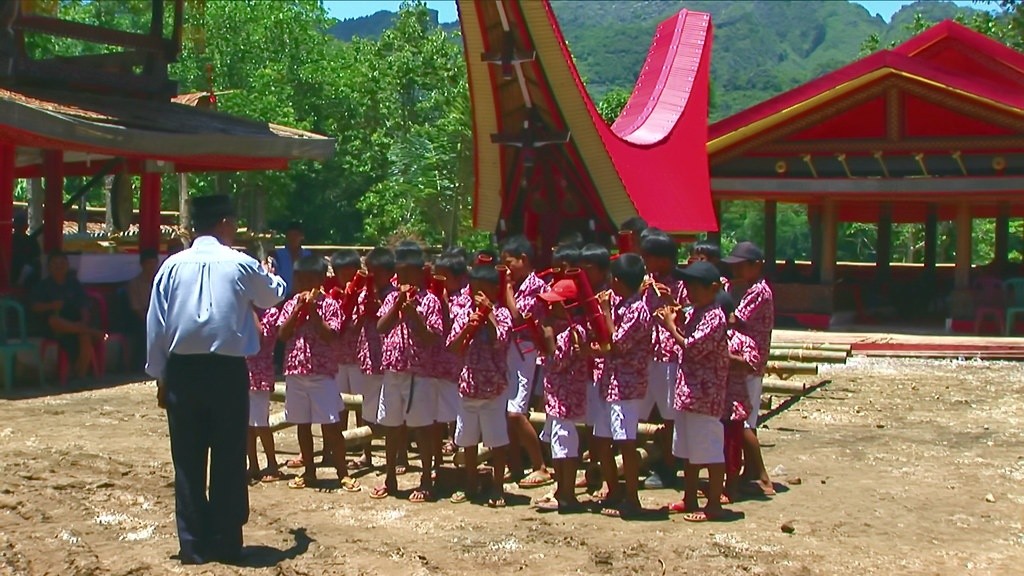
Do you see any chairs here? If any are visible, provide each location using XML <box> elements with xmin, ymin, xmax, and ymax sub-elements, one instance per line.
<box><xmin>0</xmin><ymin>287</ymin><xmax>130</xmax><ymax>395</ymax></box>
<box><xmin>973</xmin><ymin>276</ymin><xmax>1024</xmax><ymax>337</ymax></box>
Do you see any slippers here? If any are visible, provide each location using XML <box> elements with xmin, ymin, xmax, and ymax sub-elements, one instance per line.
<box><xmin>661</xmin><ymin>501</ymin><xmax>695</xmax><ymax>513</ymax></box>
<box><xmin>450</xmin><ymin>488</ymin><xmax>469</xmax><ymax>503</ymax></box>
<box><xmin>685</xmin><ymin>508</ymin><xmax>720</xmax><ymax>521</ymax></box>
<box><xmin>340</xmin><ymin>476</ymin><xmax>361</xmax><ymax>492</ymax></box>
<box><xmin>518</xmin><ymin>472</ymin><xmax>554</xmax><ymax>487</ymax></box>
<box><xmin>288</xmin><ymin>474</ymin><xmax>317</xmax><ymax>487</ymax></box>
<box><xmin>504</xmin><ymin>470</ymin><xmax>524</xmax><ymax>483</ymax></box>
<box><xmin>601</xmin><ymin>504</ymin><xmax>645</xmax><ymax>517</ymax></box>
<box><xmin>370</xmin><ymin>484</ymin><xmax>398</xmax><ymax>499</ymax></box>
<box><xmin>409</xmin><ymin>487</ymin><xmax>432</xmax><ymax>501</ymax></box>
<box><xmin>487</xmin><ymin>491</ymin><xmax>507</xmax><ymax>507</ymax></box>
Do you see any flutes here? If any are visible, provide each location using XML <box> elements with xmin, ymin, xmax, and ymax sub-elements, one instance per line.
<box><xmin>595</xmin><ymin>289</ymin><xmax>613</xmax><ymax>299</ymax></box>
<box><xmin>474</xmin><ymin>307</ymin><xmax>478</xmax><ymax>323</ymax></box>
<box><xmin>644</xmin><ymin>264</ymin><xmax>661</xmax><ymax>298</ymax></box>
<box><xmin>652</xmin><ymin>301</ymin><xmax>696</xmax><ymax>316</ymax></box>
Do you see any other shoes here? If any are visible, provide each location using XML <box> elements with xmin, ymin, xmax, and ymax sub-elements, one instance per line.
<box><xmin>535</xmin><ymin>490</ymin><xmax>575</xmax><ymax>509</ymax></box>
<box><xmin>261</xmin><ymin>470</ymin><xmax>284</xmax><ymax>482</ymax></box>
<box><xmin>442</xmin><ymin>442</ymin><xmax>453</xmax><ymax>454</ymax></box>
<box><xmin>577</xmin><ymin>461</ymin><xmax>776</xmax><ymax>501</ymax></box>
<box><xmin>286</xmin><ymin>458</ymin><xmax>304</xmax><ymax>467</ymax></box>
<box><xmin>395</xmin><ymin>460</ymin><xmax>409</xmax><ymax>474</ymax></box>
<box><xmin>347</xmin><ymin>458</ymin><xmax>371</xmax><ymax>469</ymax></box>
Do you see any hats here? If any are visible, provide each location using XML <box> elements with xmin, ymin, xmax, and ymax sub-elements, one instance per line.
<box><xmin>47</xmin><ymin>247</ymin><xmax>65</xmax><ymax>265</ymax></box>
<box><xmin>674</xmin><ymin>260</ymin><xmax>719</xmax><ymax>282</ymax></box>
<box><xmin>721</xmin><ymin>242</ymin><xmax>763</xmax><ymax>263</ymax></box>
<box><xmin>536</xmin><ymin>279</ymin><xmax>578</xmax><ymax>302</ymax></box>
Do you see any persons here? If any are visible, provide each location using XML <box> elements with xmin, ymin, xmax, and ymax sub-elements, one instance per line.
<box><xmin>346</xmin><ymin>247</ymin><xmax>412</xmax><ymax>476</ymax></box>
<box><xmin>655</xmin><ymin>260</ymin><xmax>728</xmax><ymax>521</ymax></box>
<box><xmin>534</xmin><ymin>279</ymin><xmax>589</xmax><ymax>512</ymax></box>
<box><xmin>447</xmin><ymin>265</ymin><xmax>512</xmax><ymax>508</ymax></box>
<box><xmin>41</xmin><ymin>251</ymin><xmax>110</xmax><ymax>379</ymax></box>
<box><xmin>593</xmin><ymin>253</ymin><xmax>654</xmax><ymax>517</ymax></box>
<box><xmin>721</xmin><ymin>241</ymin><xmax>778</xmax><ymax>495</ymax></box>
<box><xmin>276</xmin><ymin>254</ymin><xmax>364</xmax><ymax>494</ymax></box>
<box><xmin>368</xmin><ymin>253</ymin><xmax>443</xmax><ymax>504</ymax></box>
<box><xmin>287</xmin><ymin>251</ymin><xmax>367</xmax><ymax>470</ymax></box>
<box><xmin>146</xmin><ymin>194</ymin><xmax>287</xmax><ymax>566</ymax></box>
<box><xmin>12</xmin><ymin>214</ymin><xmax>41</xmax><ymax>292</ymax></box>
<box><xmin>246</xmin><ymin>307</ymin><xmax>286</xmax><ymax>487</ymax></box>
<box><xmin>129</xmin><ymin>248</ymin><xmax>163</xmax><ymax>320</ymax></box>
<box><xmin>271</xmin><ymin>222</ymin><xmax>312</xmax><ymax>373</ymax></box>
<box><xmin>395</xmin><ymin>215</ymin><xmax>731</xmax><ymax>488</ymax></box>
